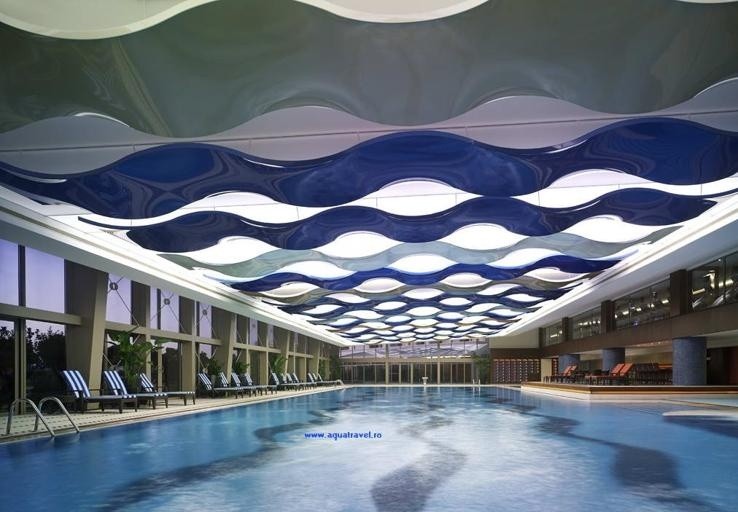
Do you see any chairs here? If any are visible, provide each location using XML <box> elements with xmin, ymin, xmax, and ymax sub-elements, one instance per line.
<box><xmin>545</xmin><ymin>361</ymin><xmax>671</xmax><ymax>385</ymax></box>
<box><xmin>60</xmin><ymin>369</ymin><xmax>339</xmax><ymax>414</ymax></box>
